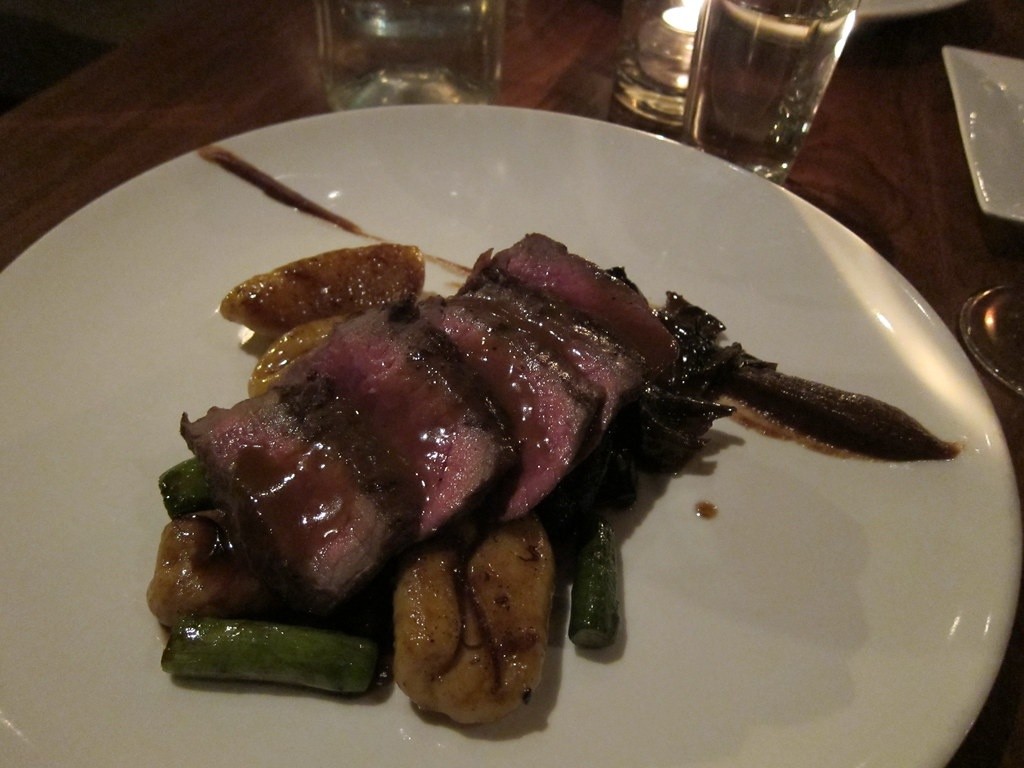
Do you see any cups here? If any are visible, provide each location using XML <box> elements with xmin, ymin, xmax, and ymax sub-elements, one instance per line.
<box><xmin>315</xmin><ymin>1</ymin><xmax>505</xmax><ymax>112</ymax></box>
<box><xmin>682</xmin><ymin>0</ymin><xmax>859</xmax><ymax>187</ymax></box>
<box><xmin>615</xmin><ymin>0</ymin><xmax>705</xmax><ymax>128</ymax></box>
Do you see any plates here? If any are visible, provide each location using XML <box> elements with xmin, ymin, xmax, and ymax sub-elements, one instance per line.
<box><xmin>943</xmin><ymin>46</ymin><xmax>1024</xmax><ymax>222</ymax></box>
<box><xmin>0</xmin><ymin>105</ymin><xmax>1019</xmax><ymax>768</ymax></box>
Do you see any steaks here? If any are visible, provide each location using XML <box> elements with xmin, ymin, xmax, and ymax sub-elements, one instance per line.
<box><xmin>179</xmin><ymin>233</ymin><xmax>678</xmax><ymax>605</ymax></box>
